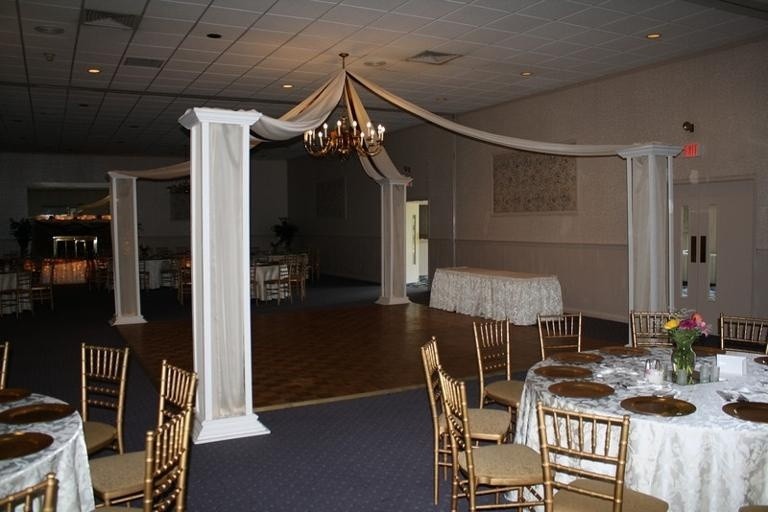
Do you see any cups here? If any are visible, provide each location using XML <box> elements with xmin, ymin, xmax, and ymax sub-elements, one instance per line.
<box><xmin>645</xmin><ymin>358</ymin><xmax>664</xmax><ymax>371</ymax></box>
<box><xmin>676</xmin><ymin>365</ymin><xmax>720</xmax><ymax>385</ymax></box>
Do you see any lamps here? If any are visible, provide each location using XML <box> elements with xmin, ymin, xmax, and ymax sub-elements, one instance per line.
<box><xmin>303</xmin><ymin>53</ymin><xmax>385</xmax><ymax>158</ymax></box>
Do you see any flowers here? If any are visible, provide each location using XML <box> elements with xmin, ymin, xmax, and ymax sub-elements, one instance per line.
<box><xmin>663</xmin><ymin>307</ymin><xmax>713</xmax><ymax>383</ymax></box>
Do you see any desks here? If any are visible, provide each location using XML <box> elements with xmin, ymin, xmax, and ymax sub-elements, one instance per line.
<box><xmin>429</xmin><ymin>266</ymin><xmax>564</xmax><ymax>326</ymax></box>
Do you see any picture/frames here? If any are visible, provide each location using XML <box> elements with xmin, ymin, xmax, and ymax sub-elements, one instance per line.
<box><xmin>314</xmin><ymin>176</ymin><xmax>348</xmax><ymax>221</ymax></box>
<box><xmin>490</xmin><ymin>138</ymin><xmax>579</xmax><ymax>217</ymax></box>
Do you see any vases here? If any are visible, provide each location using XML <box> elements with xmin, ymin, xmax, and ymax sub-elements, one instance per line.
<box><xmin>671</xmin><ymin>333</ymin><xmax>697</xmax><ymax>385</ymax></box>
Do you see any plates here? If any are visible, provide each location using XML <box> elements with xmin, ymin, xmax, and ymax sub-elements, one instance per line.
<box><xmin>548</xmin><ymin>380</ymin><xmax>615</xmax><ymax>398</ymax></box>
<box><xmin>0</xmin><ymin>432</ymin><xmax>54</xmax><ymax>459</ymax></box>
<box><xmin>534</xmin><ymin>365</ymin><xmax>594</xmax><ymax>379</ymax></box>
<box><xmin>754</xmin><ymin>357</ymin><xmax>768</xmax><ymax>365</ymax></box>
<box><xmin>621</xmin><ymin>396</ymin><xmax>697</xmax><ymax>417</ymax></box>
<box><xmin>549</xmin><ymin>350</ymin><xmax>604</xmax><ymax>364</ymax></box>
<box><xmin>0</xmin><ymin>403</ymin><xmax>76</xmax><ymax>423</ymax></box>
<box><xmin>722</xmin><ymin>402</ymin><xmax>768</xmax><ymax>422</ymax></box>
<box><xmin>0</xmin><ymin>388</ymin><xmax>32</xmax><ymax>403</ymax></box>
<box><xmin>692</xmin><ymin>345</ymin><xmax>726</xmax><ymax>357</ymax></box>
<box><xmin>600</xmin><ymin>346</ymin><xmax>651</xmax><ymax>358</ymax></box>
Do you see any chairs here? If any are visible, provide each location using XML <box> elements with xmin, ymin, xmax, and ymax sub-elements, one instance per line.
<box><xmin>89</xmin><ymin>402</ymin><xmax>192</xmax><ymax>512</ymax></box>
<box><xmin>473</xmin><ymin>315</ymin><xmax>525</xmax><ymax>443</ymax></box>
<box><xmin>537</xmin><ymin>312</ymin><xmax>582</xmax><ymax>361</ymax></box>
<box><xmin>631</xmin><ymin>308</ymin><xmax>673</xmax><ymax>348</ymax></box>
<box><xmin>0</xmin><ymin>472</ymin><xmax>57</xmax><ymax>512</ymax></box>
<box><xmin>81</xmin><ymin>342</ymin><xmax>130</xmax><ymax>455</ymax></box>
<box><xmin>720</xmin><ymin>312</ymin><xmax>768</xmax><ymax>354</ymax></box>
<box><xmin>438</xmin><ymin>364</ymin><xmax>555</xmax><ymax>512</ymax></box>
<box><xmin>0</xmin><ymin>341</ymin><xmax>9</xmax><ymax>389</ymax></box>
<box><xmin>421</xmin><ymin>336</ymin><xmax>512</xmax><ymax>506</ymax></box>
<box><xmin>90</xmin><ymin>359</ymin><xmax>196</xmax><ymax>508</ymax></box>
<box><xmin>535</xmin><ymin>401</ymin><xmax>668</xmax><ymax>512</ymax></box>
